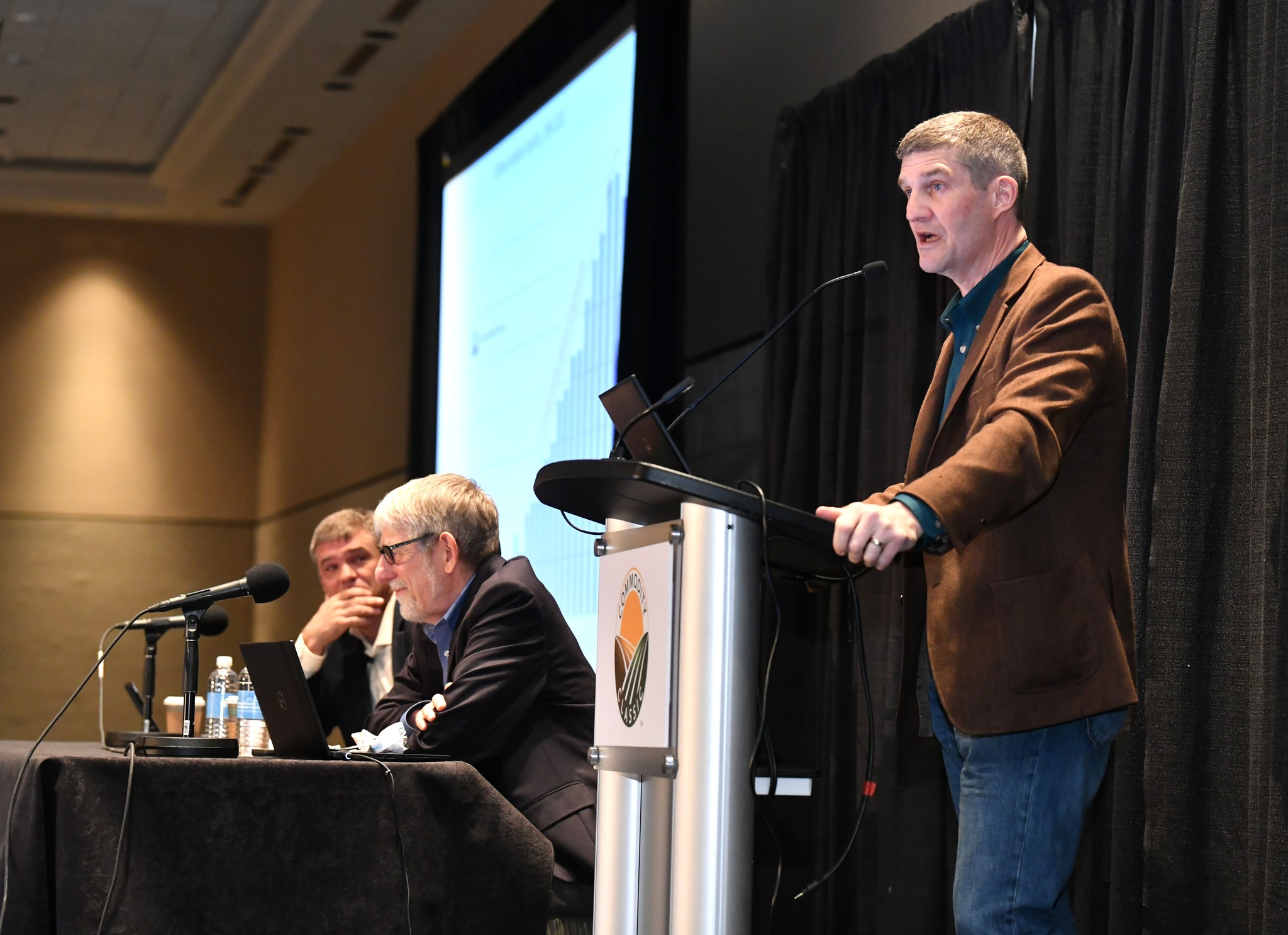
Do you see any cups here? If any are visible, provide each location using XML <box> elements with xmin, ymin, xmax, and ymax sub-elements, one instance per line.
<box><xmin>163</xmin><ymin>696</ymin><xmax>206</xmax><ymax>738</ymax></box>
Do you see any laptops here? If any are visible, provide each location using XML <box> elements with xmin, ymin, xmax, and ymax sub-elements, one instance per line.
<box><xmin>598</xmin><ymin>374</ymin><xmax>694</xmax><ymax>477</ymax></box>
<box><xmin>237</xmin><ymin>640</ymin><xmax>450</xmax><ymax>762</ymax></box>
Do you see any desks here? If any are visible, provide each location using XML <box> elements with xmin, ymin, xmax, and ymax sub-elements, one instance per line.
<box><xmin>0</xmin><ymin>742</ymin><xmax>556</xmax><ymax>935</ymax></box>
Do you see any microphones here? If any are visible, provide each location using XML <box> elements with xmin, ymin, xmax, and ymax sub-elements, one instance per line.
<box><xmin>662</xmin><ymin>258</ymin><xmax>890</xmax><ymax>432</ymax></box>
<box><xmin>102</xmin><ymin>603</ymin><xmax>229</xmax><ymax>746</ymax></box>
<box><xmin>130</xmin><ymin>561</ymin><xmax>292</xmax><ymax>758</ymax></box>
<box><xmin>609</xmin><ymin>376</ymin><xmax>694</xmax><ymax>460</ymax></box>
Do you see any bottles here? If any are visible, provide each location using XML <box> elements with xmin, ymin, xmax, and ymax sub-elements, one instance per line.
<box><xmin>235</xmin><ymin>666</ymin><xmax>269</xmax><ymax>756</ymax></box>
<box><xmin>205</xmin><ymin>656</ymin><xmax>239</xmax><ymax>739</ymax></box>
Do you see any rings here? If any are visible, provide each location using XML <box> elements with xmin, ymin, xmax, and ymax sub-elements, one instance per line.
<box><xmin>869</xmin><ymin>536</ymin><xmax>887</xmax><ymax>552</ymax></box>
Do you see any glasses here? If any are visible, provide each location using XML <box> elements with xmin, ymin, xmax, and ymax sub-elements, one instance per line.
<box><xmin>381</xmin><ymin>531</ymin><xmax>437</xmax><ymax>564</ymax></box>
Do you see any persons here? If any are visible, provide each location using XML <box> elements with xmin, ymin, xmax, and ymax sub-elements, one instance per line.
<box><xmin>360</xmin><ymin>473</ymin><xmax>593</xmax><ymax>934</ymax></box>
<box><xmin>810</xmin><ymin>106</ymin><xmax>1138</xmax><ymax>935</ymax></box>
<box><xmin>294</xmin><ymin>506</ymin><xmax>414</xmax><ymax>749</ymax></box>
